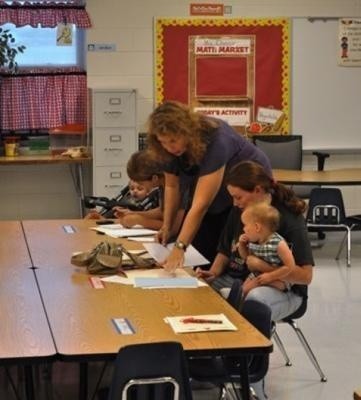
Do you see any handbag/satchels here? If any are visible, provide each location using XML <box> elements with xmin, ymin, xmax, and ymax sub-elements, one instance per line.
<box><xmin>87</xmin><ymin>240</ymin><xmax>122</xmax><ymax>274</ymax></box>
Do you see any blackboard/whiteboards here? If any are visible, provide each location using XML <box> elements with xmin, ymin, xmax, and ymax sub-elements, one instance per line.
<box><xmin>290</xmin><ymin>16</ymin><xmax>361</xmax><ymax>154</ymax></box>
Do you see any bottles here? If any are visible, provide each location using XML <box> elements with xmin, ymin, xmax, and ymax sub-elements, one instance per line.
<box><xmin>5</xmin><ymin>143</ymin><xmax>19</xmax><ymax>156</ymax></box>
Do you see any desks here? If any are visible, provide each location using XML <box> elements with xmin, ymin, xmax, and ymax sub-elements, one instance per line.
<box><xmin>20</xmin><ymin>217</ymin><xmax>272</xmax><ymax>399</ymax></box>
<box><xmin>0</xmin><ymin>150</ymin><xmax>92</xmax><ymax>219</ymax></box>
<box><xmin>272</xmin><ymin>168</ymin><xmax>361</xmax><ymax>231</ymax></box>
<box><xmin>1</xmin><ymin>218</ymin><xmax>57</xmax><ymax>399</ymax></box>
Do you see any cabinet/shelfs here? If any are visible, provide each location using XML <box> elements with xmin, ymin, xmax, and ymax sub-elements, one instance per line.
<box><xmin>92</xmin><ymin>89</ymin><xmax>139</xmax><ymax>213</ymax></box>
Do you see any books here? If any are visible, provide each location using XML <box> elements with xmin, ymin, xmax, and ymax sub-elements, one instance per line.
<box><xmin>165</xmin><ymin>312</ymin><xmax>239</xmax><ymax>336</ymax></box>
<box><xmin>141</xmin><ymin>240</ymin><xmax>214</xmax><ymax>269</ymax></box>
<box><xmin>91</xmin><ymin>221</ymin><xmax>161</xmax><ymax>240</ymax></box>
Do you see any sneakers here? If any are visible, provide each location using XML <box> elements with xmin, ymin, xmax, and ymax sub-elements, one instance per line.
<box><xmin>190</xmin><ymin>379</ymin><xmax>216</xmax><ymax>389</ymax></box>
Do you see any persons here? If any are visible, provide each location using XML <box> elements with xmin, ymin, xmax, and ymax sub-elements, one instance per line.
<box><xmin>190</xmin><ymin>159</ymin><xmax>317</xmax><ymax>342</ymax></box>
<box><xmin>145</xmin><ymin>97</ymin><xmax>275</xmax><ymax>278</ymax></box>
<box><xmin>234</xmin><ymin>202</ymin><xmax>298</xmax><ymax>296</ymax></box>
<box><xmin>111</xmin><ymin>149</ymin><xmax>199</xmax><ymax>239</ymax></box>
<box><xmin>84</xmin><ymin>178</ymin><xmax>154</xmax><ymax>222</ymax></box>
<box><xmin>341</xmin><ymin>37</ymin><xmax>350</xmax><ymax>58</ymax></box>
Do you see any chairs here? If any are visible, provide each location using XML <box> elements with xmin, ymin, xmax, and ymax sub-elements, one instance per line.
<box><xmin>190</xmin><ymin>298</ymin><xmax>272</xmax><ymax>399</ymax></box>
<box><xmin>253</xmin><ymin>135</ymin><xmax>330</xmax><ymax>239</ymax></box>
<box><xmin>96</xmin><ymin>341</ymin><xmax>194</xmax><ymax>398</ymax></box>
<box><xmin>272</xmin><ymin>285</ymin><xmax>328</xmax><ymax>383</ymax></box>
<box><xmin>306</xmin><ymin>188</ymin><xmax>351</xmax><ymax>267</ymax></box>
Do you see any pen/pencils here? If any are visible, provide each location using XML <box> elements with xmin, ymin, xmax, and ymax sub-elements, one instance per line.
<box><xmin>184</xmin><ymin>319</ymin><xmax>222</xmax><ymax>323</ymax></box>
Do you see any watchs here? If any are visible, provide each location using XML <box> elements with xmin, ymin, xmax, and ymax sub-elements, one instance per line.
<box><xmin>175</xmin><ymin>239</ymin><xmax>188</xmax><ymax>252</ymax></box>
